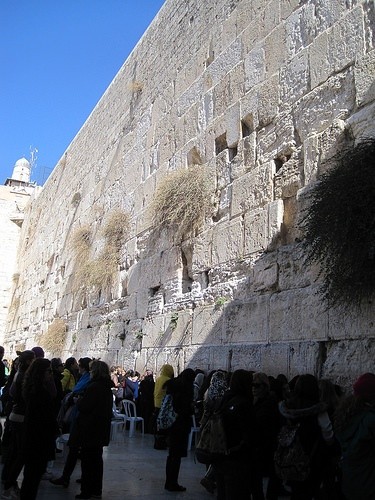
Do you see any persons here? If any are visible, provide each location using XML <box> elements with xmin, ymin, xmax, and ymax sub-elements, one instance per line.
<box><xmin>0</xmin><ymin>357</ymin><xmax>60</xmax><ymax>500</ymax></box>
<box><xmin>110</xmin><ymin>365</ymin><xmax>344</xmax><ymax>495</ymax></box>
<box><xmin>213</xmin><ymin>369</ymin><xmax>259</xmax><ymax>500</ymax></box>
<box><xmin>278</xmin><ymin>374</ymin><xmax>334</xmax><ymax>500</ymax></box>
<box><xmin>162</xmin><ymin>368</ymin><xmax>195</xmax><ymax>492</ymax></box>
<box><xmin>333</xmin><ymin>373</ymin><xmax>375</xmax><ymax>500</ymax></box>
<box><xmin>0</xmin><ymin>346</ymin><xmax>93</xmax><ymax>487</ymax></box>
<box><xmin>73</xmin><ymin>360</ymin><xmax>113</xmax><ymax>500</ymax></box>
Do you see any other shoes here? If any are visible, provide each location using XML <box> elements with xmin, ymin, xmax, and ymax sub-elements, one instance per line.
<box><xmin>11</xmin><ymin>486</ymin><xmax>20</xmax><ymax>500</ymax></box>
<box><xmin>76</xmin><ymin>478</ymin><xmax>82</xmax><ymax>483</ymax></box>
<box><xmin>48</xmin><ymin>476</ymin><xmax>71</xmax><ymax>489</ymax></box>
<box><xmin>164</xmin><ymin>484</ymin><xmax>168</xmax><ymax>490</ymax></box>
<box><xmin>41</xmin><ymin>470</ymin><xmax>55</xmax><ymax>480</ymax></box>
<box><xmin>168</xmin><ymin>484</ymin><xmax>186</xmax><ymax>491</ymax></box>
<box><xmin>75</xmin><ymin>491</ymin><xmax>102</xmax><ymax>500</ymax></box>
<box><xmin>0</xmin><ymin>488</ymin><xmax>17</xmax><ymax>500</ymax></box>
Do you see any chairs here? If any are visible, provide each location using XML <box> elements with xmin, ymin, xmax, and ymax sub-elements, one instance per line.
<box><xmin>186</xmin><ymin>415</ymin><xmax>201</xmax><ymax>451</ymax></box>
<box><xmin>109</xmin><ymin>394</ymin><xmax>144</xmax><ymax>438</ymax></box>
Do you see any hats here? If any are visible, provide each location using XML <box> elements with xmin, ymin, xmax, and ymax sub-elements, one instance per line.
<box><xmin>353</xmin><ymin>373</ymin><xmax>375</xmax><ymax>394</ymax></box>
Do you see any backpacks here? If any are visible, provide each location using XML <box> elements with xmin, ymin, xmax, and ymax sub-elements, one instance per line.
<box><xmin>274</xmin><ymin>421</ymin><xmax>311</xmax><ymax>482</ymax></box>
<box><xmin>194</xmin><ymin>395</ymin><xmax>245</xmax><ymax>465</ymax></box>
<box><xmin>157</xmin><ymin>395</ymin><xmax>178</xmax><ymax>430</ymax></box>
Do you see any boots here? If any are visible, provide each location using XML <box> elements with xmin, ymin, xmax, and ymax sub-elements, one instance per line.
<box><xmin>200</xmin><ymin>464</ymin><xmax>216</xmax><ymax>494</ymax></box>
